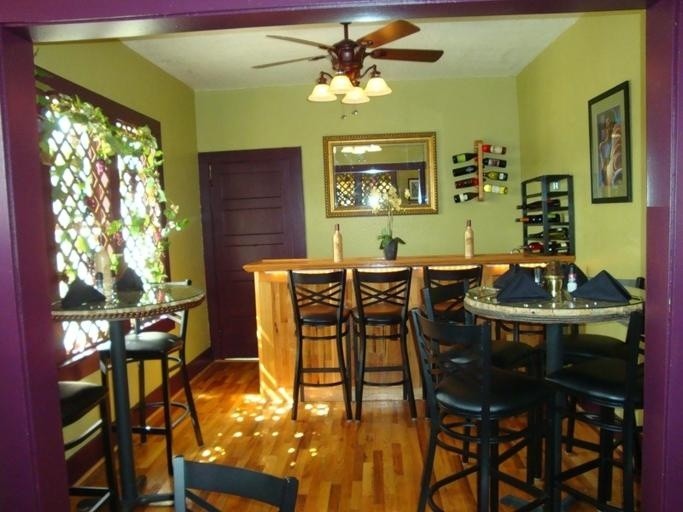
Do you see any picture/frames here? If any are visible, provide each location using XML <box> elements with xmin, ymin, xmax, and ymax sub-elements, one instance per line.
<box><xmin>408</xmin><ymin>177</ymin><xmax>420</xmax><ymax>201</ymax></box>
<box><xmin>586</xmin><ymin>79</ymin><xmax>634</xmax><ymax>205</ymax></box>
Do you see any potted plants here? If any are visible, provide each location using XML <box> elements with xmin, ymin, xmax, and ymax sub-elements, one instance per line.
<box><xmin>378</xmin><ymin>230</ymin><xmax>405</xmax><ymax>260</ymax></box>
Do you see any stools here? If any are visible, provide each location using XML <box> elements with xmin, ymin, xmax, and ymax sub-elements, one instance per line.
<box><xmin>54</xmin><ymin>378</ymin><xmax>121</xmax><ymax>511</ymax></box>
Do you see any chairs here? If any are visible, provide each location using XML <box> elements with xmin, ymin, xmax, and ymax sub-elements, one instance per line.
<box><xmin>96</xmin><ymin>277</ymin><xmax>203</xmax><ymax>476</ymax></box>
<box><xmin>172</xmin><ymin>451</ymin><xmax>298</xmax><ymax>511</ymax></box>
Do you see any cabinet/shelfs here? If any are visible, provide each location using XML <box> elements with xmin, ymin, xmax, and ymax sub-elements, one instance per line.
<box><xmin>522</xmin><ymin>172</ymin><xmax>576</xmax><ymax>256</ymax></box>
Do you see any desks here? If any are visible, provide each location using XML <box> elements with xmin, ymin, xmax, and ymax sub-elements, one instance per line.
<box><xmin>45</xmin><ymin>281</ymin><xmax>208</xmax><ymax>511</ymax></box>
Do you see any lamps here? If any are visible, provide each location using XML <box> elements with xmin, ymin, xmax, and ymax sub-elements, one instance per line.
<box><xmin>306</xmin><ymin>56</ymin><xmax>392</xmax><ymax>104</ymax></box>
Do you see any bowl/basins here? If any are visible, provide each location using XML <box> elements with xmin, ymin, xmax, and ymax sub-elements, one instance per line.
<box><xmin>116</xmin><ymin>291</ymin><xmax>143</xmax><ymax>306</ymax></box>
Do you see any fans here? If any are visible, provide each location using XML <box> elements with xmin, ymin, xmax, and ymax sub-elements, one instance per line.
<box><xmin>248</xmin><ymin>15</ymin><xmax>445</xmax><ymax>69</ymax></box>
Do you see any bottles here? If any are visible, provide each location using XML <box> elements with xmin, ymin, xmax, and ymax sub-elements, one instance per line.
<box><xmin>332</xmin><ymin>224</ymin><xmax>343</xmax><ymax>263</ymax></box>
<box><xmin>514</xmin><ymin>199</ymin><xmax>569</xmax><ymax>255</ymax></box>
<box><xmin>566</xmin><ymin>266</ymin><xmax>577</xmax><ymax>294</ymax></box>
<box><xmin>463</xmin><ymin>219</ymin><xmax>474</xmax><ymax>258</ymax></box>
<box><xmin>93</xmin><ymin>243</ymin><xmax>111</xmax><ymax>294</ymax></box>
<box><xmin>452</xmin><ymin>143</ymin><xmax>508</xmax><ymax>204</ymax></box>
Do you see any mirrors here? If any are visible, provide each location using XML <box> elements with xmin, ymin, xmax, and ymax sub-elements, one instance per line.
<box><xmin>322</xmin><ymin>131</ymin><xmax>439</xmax><ymax>219</ymax></box>
